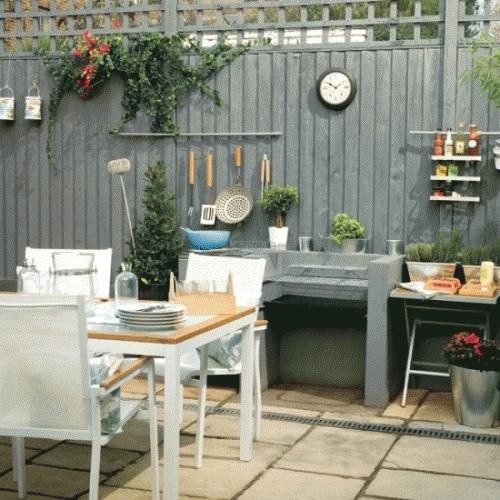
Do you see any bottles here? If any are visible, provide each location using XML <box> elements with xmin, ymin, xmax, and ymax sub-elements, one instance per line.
<box><xmin>432</xmin><ymin>122</ymin><xmax>478</xmax><ymax>199</ymax></box>
<box><xmin>114</xmin><ymin>261</ymin><xmax>137</xmax><ymax>311</ymax></box>
<box><xmin>454</xmin><ymin>253</ymin><xmax>467</xmax><ymax>285</ymax></box>
<box><xmin>15</xmin><ymin>255</ymin><xmax>40</xmax><ymax>296</ymax></box>
<box><xmin>478</xmin><ymin>259</ymin><xmax>495</xmax><ymax>292</ymax></box>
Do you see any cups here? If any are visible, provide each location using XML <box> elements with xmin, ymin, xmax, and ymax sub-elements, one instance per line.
<box><xmin>386</xmin><ymin>240</ymin><xmax>404</xmax><ymax>258</ymax></box>
<box><xmin>296</xmin><ymin>237</ymin><xmax>313</xmax><ymax>251</ymax></box>
<box><xmin>49</xmin><ymin>250</ymin><xmax>98</xmax><ymax>316</ymax></box>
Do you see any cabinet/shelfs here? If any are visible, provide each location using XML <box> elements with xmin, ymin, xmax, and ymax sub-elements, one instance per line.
<box><xmin>428</xmin><ymin>154</ymin><xmax>483</xmax><ymax>206</ymax></box>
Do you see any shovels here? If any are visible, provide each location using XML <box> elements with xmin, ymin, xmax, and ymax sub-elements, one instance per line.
<box><xmin>199</xmin><ymin>149</ymin><xmax>216</xmax><ymax>225</ymax></box>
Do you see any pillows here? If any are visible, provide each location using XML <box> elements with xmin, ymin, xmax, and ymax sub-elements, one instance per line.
<box><xmin>14</xmin><ymin>265</ymin><xmax>75</xmax><ymax>293</ymax></box>
<box><xmin>87</xmin><ymin>359</ymin><xmax>123</xmax><ymax>436</ymax></box>
<box><xmin>196</xmin><ymin>328</ymin><xmax>243</xmax><ymax>370</ymax></box>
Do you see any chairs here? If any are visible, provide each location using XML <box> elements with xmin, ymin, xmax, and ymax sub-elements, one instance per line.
<box><xmin>22</xmin><ymin>243</ymin><xmax>113</xmax><ymax>300</ymax></box>
<box><xmin>1</xmin><ymin>292</ymin><xmax>162</xmax><ymax>500</ymax></box>
<box><xmin>121</xmin><ymin>252</ymin><xmax>274</xmax><ymax>468</ymax></box>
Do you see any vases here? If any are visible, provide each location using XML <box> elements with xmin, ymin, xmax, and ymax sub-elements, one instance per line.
<box><xmin>448</xmin><ymin>363</ymin><xmax>500</xmax><ymax>429</ymax></box>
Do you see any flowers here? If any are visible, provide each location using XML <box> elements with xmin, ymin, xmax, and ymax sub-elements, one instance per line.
<box><xmin>436</xmin><ymin>326</ymin><xmax>500</xmax><ymax>371</ymax></box>
<box><xmin>34</xmin><ymin>28</ymin><xmax>253</xmax><ymax>175</ymax></box>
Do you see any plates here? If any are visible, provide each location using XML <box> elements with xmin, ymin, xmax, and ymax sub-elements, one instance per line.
<box><xmin>115</xmin><ymin>302</ymin><xmax>185</xmax><ymax>330</ymax></box>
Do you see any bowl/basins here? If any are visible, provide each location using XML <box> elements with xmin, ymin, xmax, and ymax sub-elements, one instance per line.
<box><xmin>188</xmin><ymin>231</ymin><xmax>230</xmax><ymax>248</ymax></box>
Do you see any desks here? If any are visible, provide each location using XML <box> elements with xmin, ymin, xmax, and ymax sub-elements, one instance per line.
<box><xmin>388</xmin><ymin>286</ymin><xmax>500</xmax><ymax>408</ymax></box>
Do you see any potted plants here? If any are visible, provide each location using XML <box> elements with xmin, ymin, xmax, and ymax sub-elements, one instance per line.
<box><xmin>255</xmin><ymin>182</ymin><xmax>297</xmax><ymax>249</ymax></box>
<box><xmin>407</xmin><ymin>228</ymin><xmax>459</xmax><ymax>281</ymax></box>
<box><xmin>458</xmin><ymin>238</ymin><xmax>500</xmax><ymax>285</ymax></box>
<box><xmin>330</xmin><ymin>213</ymin><xmax>370</xmax><ymax>253</ymax></box>
<box><xmin>114</xmin><ymin>158</ymin><xmax>185</xmax><ymax>300</ymax></box>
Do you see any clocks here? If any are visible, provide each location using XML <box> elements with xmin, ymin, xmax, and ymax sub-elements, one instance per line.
<box><xmin>317</xmin><ymin>68</ymin><xmax>356</xmax><ymax>111</ymax></box>
